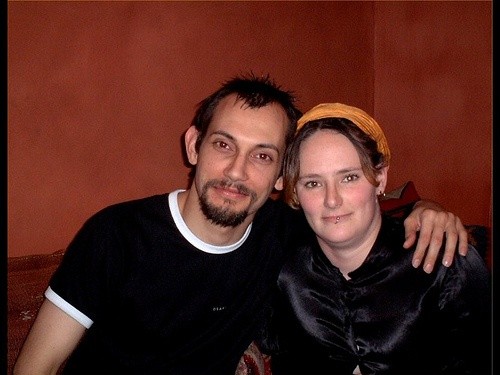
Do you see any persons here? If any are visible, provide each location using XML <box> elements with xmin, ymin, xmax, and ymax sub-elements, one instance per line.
<box><xmin>270</xmin><ymin>104</ymin><xmax>493</xmax><ymax>375</ymax></box>
<box><xmin>14</xmin><ymin>77</ymin><xmax>469</xmax><ymax>375</ymax></box>
